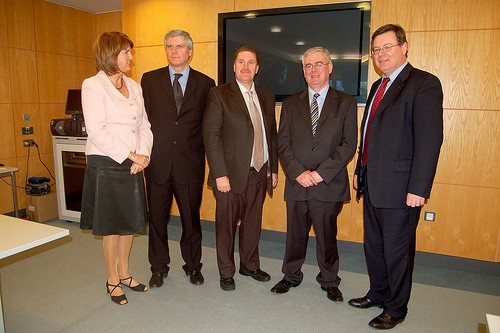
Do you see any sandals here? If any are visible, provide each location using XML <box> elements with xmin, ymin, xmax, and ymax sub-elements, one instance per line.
<box><xmin>119</xmin><ymin>276</ymin><xmax>148</xmax><ymax>292</ymax></box>
<box><xmin>105</xmin><ymin>279</ymin><xmax>129</xmax><ymax>306</ymax></box>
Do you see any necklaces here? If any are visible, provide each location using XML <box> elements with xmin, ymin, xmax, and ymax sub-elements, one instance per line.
<box><xmin>116</xmin><ymin>77</ymin><xmax>123</xmax><ymax>89</ymax></box>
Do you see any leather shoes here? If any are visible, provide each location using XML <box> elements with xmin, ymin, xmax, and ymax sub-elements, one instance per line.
<box><xmin>148</xmin><ymin>272</ymin><xmax>168</xmax><ymax>289</ymax></box>
<box><xmin>184</xmin><ymin>269</ymin><xmax>205</xmax><ymax>286</ymax></box>
<box><xmin>219</xmin><ymin>276</ymin><xmax>236</xmax><ymax>291</ymax></box>
<box><xmin>320</xmin><ymin>285</ymin><xmax>345</xmax><ymax>303</ymax></box>
<box><xmin>347</xmin><ymin>295</ymin><xmax>383</xmax><ymax>309</ymax></box>
<box><xmin>368</xmin><ymin>311</ymin><xmax>405</xmax><ymax>330</ymax></box>
<box><xmin>270</xmin><ymin>278</ymin><xmax>297</xmax><ymax>294</ymax></box>
<box><xmin>238</xmin><ymin>268</ymin><xmax>272</xmax><ymax>282</ymax></box>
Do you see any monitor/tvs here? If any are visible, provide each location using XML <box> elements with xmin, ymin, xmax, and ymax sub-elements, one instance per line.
<box><xmin>217</xmin><ymin>2</ymin><xmax>372</xmax><ymax>108</ymax></box>
<box><xmin>65</xmin><ymin>87</ymin><xmax>82</xmax><ymax>119</ymax></box>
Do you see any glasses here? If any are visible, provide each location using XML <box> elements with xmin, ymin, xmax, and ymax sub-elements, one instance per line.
<box><xmin>371</xmin><ymin>44</ymin><xmax>400</xmax><ymax>56</ymax></box>
<box><xmin>303</xmin><ymin>62</ymin><xmax>330</xmax><ymax>71</ymax></box>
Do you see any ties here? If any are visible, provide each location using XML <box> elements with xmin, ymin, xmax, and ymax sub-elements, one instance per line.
<box><xmin>246</xmin><ymin>91</ymin><xmax>265</xmax><ymax>172</ymax></box>
<box><xmin>362</xmin><ymin>76</ymin><xmax>391</xmax><ymax>167</ymax></box>
<box><xmin>310</xmin><ymin>92</ymin><xmax>321</xmax><ymax>137</ymax></box>
<box><xmin>171</xmin><ymin>72</ymin><xmax>184</xmax><ymax>117</ymax></box>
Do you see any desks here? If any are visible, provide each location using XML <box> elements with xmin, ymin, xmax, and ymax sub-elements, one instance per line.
<box><xmin>0</xmin><ymin>213</ymin><xmax>69</xmax><ymax>333</ymax></box>
<box><xmin>0</xmin><ymin>165</ymin><xmax>19</xmax><ymax>219</ymax></box>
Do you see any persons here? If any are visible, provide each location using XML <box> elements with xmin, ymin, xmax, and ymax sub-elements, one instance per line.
<box><xmin>348</xmin><ymin>23</ymin><xmax>443</xmax><ymax>329</ymax></box>
<box><xmin>80</xmin><ymin>32</ymin><xmax>153</xmax><ymax>305</ymax></box>
<box><xmin>271</xmin><ymin>46</ymin><xmax>358</xmax><ymax>302</ymax></box>
<box><xmin>141</xmin><ymin>29</ymin><xmax>216</xmax><ymax>290</ymax></box>
<box><xmin>202</xmin><ymin>45</ymin><xmax>279</xmax><ymax>291</ymax></box>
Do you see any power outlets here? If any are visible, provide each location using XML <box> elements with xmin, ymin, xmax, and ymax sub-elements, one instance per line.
<box><xmin>23</xmin><ymin>139</ymin><xmax>35</xmax><ymax>147</ymax></box>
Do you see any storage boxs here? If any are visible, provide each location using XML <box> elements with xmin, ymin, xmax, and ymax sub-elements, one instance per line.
<box><xmin>24</xmin><ymin>194</ymin><xmax>58</xmax><ymax>223</ymax></box>
